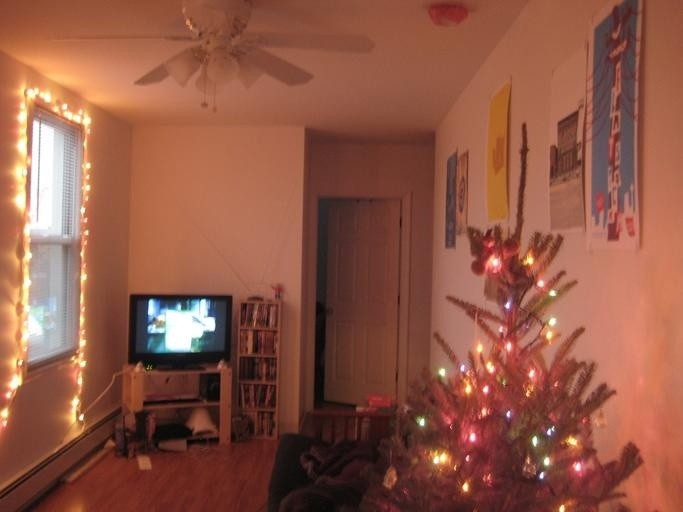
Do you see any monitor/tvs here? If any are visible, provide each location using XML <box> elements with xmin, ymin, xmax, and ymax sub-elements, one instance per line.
<box><xmin>128</xmin><ymin>293</ymin><xmax>232</xmax><ymax>370</ymax></box>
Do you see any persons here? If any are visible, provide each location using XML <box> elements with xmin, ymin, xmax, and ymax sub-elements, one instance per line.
<box><xmin>278</xmin><ymin>432</ymin><xmax>397</xmax><ymax>512</ymax></box>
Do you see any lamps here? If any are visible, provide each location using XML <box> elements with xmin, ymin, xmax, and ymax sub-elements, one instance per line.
<box><xmin>202</xmin><ymin>35</ymin><xmax>239</xmax><ymax>83</ymax></box>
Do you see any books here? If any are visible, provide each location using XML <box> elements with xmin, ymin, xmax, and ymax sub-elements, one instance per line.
<box><xmin>240</xmin><ymin>303</ymin><xmax>277</xmax><ymax>437</ymax></box>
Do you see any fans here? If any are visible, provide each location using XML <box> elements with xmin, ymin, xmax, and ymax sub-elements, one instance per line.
<box><xmin>53</xmin><ymin>1</ymin><xmax>376</xmax><ymax>113</ymax></box>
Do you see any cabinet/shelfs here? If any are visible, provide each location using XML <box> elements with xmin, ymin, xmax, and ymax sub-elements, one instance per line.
<box><xmin>237</xmin><ymin>300</ymin><xmax>280</xmax><ymax>441</ymax></box>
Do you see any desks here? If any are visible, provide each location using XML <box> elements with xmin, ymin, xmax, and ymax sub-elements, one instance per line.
<box><xmin>123</xmin><ymin>365</ymin><xmax>232</xmax><ymax>450</ymax></box>
<box><xmin>314</xmin><ymin>410</ymin><xmax>393</xmax><ymax>443</ymax></box>
